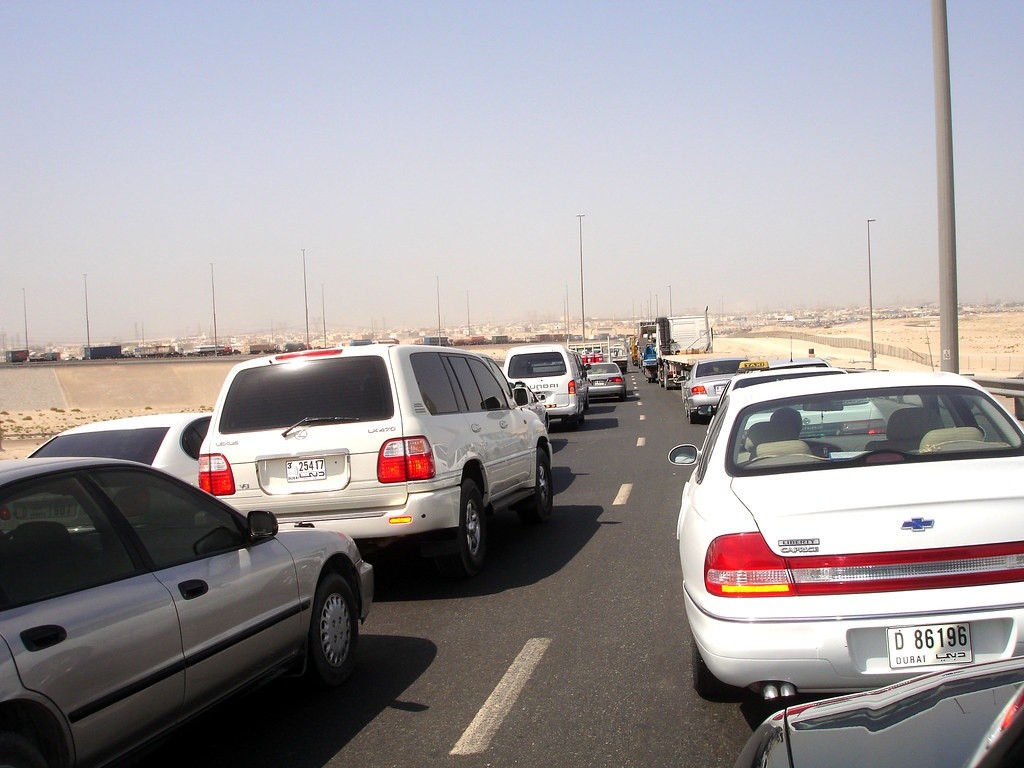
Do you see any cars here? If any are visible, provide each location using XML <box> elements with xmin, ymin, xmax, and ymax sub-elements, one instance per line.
<box><xmin>695</xmin><ymin>359</ymin><xmax>888</xmax><ymax>450</ymax></box>
<box><xmin>565</xmin><ymin>304</ymin><xmax>831</xmax><ymax>425</ymax></box>
<box><xmin>730</xmin><ymin>654</ymin><xmax>1023</xmax><ymax>768</ymax></box>
<box><xmin>1</xmin><ymin>409</ymin><xmax>216</xmax><ymax>545</ymax></box>
<box><xmin>665</xmin><ymin>368</ymin><xmax>1023</xmax><ymax>703</ymax></box>
<box><xmin>1</xmin><ymin>453</ymin><xmax>375</xmax><ymax>767</ymax></box>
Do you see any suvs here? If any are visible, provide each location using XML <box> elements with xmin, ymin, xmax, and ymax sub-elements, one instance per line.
<box><xmin>196</xmin><ymin>342</ymin><xmax>557</xmax><ymax>584</ymax></box>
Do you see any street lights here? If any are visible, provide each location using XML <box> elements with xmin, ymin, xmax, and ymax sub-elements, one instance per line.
<box><xmin>866</xmin><ymin>218</ymin><xmax>878</xmax><ymax>369</ymax></box>
<box><xmin>83</xmin><ymin>273</ymin><xmax>91</xmax><ymax>359</ymax></box>
<box><xmin>209</xmin><ymin>262</ymin><xmax>218</xmax><ymax>356</ymax></box>
<box><xmin>576</xmin><ymin>211</ymin><xmax>587</xmax><ymax>341</ymax></box>
<box><xmin>300</xmin><ymin>248</ymin><xmax>310</xmax><ymax>350</ymax></box>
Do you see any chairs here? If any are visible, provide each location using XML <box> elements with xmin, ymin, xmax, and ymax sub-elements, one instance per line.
<box><xmin>743</xmin><ymin>422</ymin><xmax>824</xmax><ymax>461</ymax></box>
<box><xmin>865</xmin><ymin>408</ymin><xmax>943</xmax><ymax>452</ymax></box>
<box><xmin>15</xmin><ymin>521</ymin><xmax>73</xmax><ymax>555</ymax></box>
<box><xmin>920</xmin><ymin>427</ymin><xmax>984</xmax><ymax>452</ymax></box>
<box><xmin>756</xmin><ymin>440</ymin><xmax>812</xmax><ymax>457</ymax></box>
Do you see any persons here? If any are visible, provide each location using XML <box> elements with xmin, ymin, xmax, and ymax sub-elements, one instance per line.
<box><xmin>749</xmin><ymin>408</ymin><xmax>802</xmax><ymax>459</ymax></box>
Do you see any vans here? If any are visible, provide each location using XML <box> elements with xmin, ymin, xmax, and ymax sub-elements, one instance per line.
<box><xmin>502</xmin><ymin>342</ymin><xmax>591</xmax><ymax>427</ymax></box>
